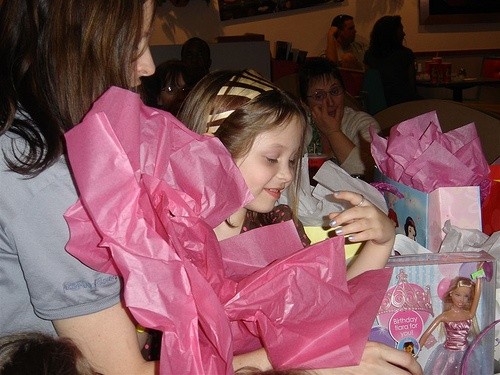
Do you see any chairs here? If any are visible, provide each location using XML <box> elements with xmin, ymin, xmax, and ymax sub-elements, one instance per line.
<box><xmin>313</xmin><ymin>53</ymin><xmax>366</xmax><ymax>110</ymax></box>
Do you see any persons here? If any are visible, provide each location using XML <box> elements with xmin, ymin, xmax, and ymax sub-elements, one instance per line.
<box><xmin>0</xmin><ymin>0</ymin><xmax>425</xmax><ymax>375</ymax></box>
<box><xmin>420</xmin><ymin>262</ymin><xmax>485</xmax><ymax>375</ymax></box>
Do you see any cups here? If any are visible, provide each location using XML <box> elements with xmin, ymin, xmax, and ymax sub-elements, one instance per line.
<box><xmin>421</xmin><ymin>56</ymin><xmax>451</xmax><ymax>83</ymax></box>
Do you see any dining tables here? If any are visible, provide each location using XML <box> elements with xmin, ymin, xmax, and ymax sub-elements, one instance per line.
<box><xmin>416</xmin><ymin>75</ymin><xmax>500</xmax><ymax>102</ymax></box>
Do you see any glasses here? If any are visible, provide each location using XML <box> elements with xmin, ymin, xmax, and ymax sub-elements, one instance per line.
<box><xmin>307</xmin><ymin>86</ymin><xmax>344</xmax><ymax>100</ymax></box>
<box><xmin>339</xmin><ymin>15</ymin><xmax>343</xmax><ymax>19</ymax></box>
<box><xmin>161</xmin><ymin>80</ymin><xmax>192</xmax><ymax>96</ymax></box>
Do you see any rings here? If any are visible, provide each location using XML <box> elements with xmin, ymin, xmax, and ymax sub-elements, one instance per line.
<box><xmin>354</xmin><ymin>193</ymin><xmax>365</xmax><ymax>207</ymax></box>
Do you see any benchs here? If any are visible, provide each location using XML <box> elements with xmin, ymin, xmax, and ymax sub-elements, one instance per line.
<box><xmin>462</xmin><ymin>57</ymin><xmax>500</xmax><ymax>118</ymax></box>
<box><xmin>371</xmin><ymin>99</ymin><xmax>500</xmax><ymax>163</ymax></box>
